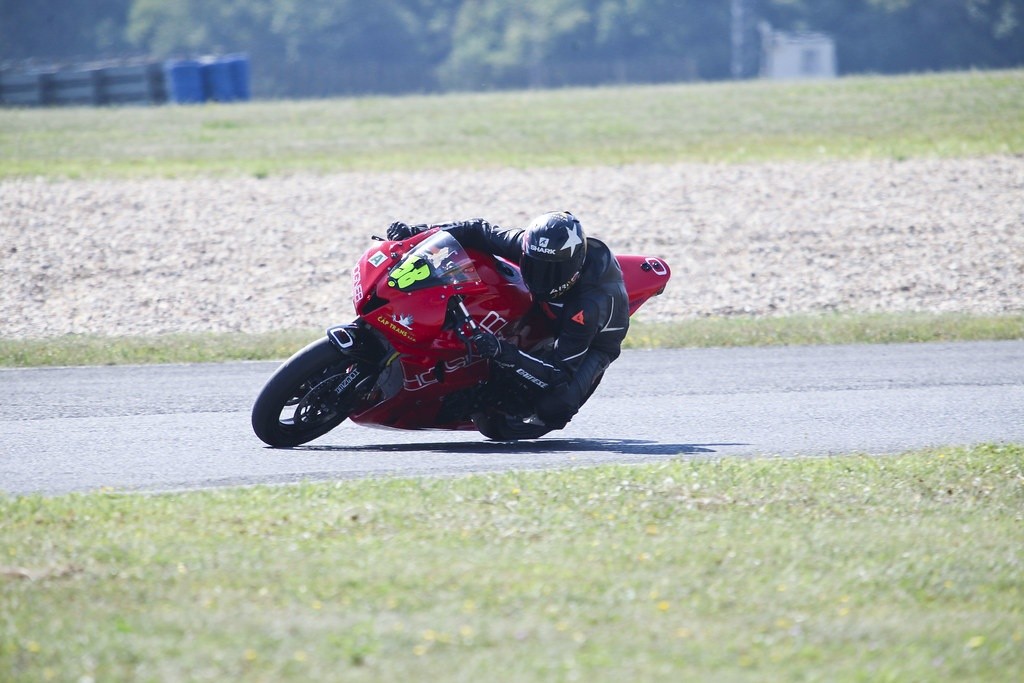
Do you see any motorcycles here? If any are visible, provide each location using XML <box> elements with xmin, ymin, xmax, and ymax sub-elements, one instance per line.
<box><xmin>250</xmin><ymin>225</ymin><xmax>672</xmax><ymax>449</ymax></box>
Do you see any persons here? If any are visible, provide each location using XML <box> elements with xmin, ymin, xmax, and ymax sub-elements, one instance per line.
<box><xmin>385</xmin><ymin>209</ymin><xmax>631</xmax><ymax>427</ymax></box>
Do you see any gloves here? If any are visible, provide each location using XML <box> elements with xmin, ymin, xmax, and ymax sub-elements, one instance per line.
<box><xmin>474</xmin><ymin>333</ymin><xmax>500</xmax><ymax>359</ymax></box>
<box><xmin>388</xmin><ymin>221</ymin><xmax>414</xmax><ymax>241</ymax></box>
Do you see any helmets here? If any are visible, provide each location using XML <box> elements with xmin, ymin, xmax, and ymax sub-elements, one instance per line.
<box><xmin>520</xmin><ymin>211</ymin><xmax>587</xmax><ymax>301</ymax></box>
